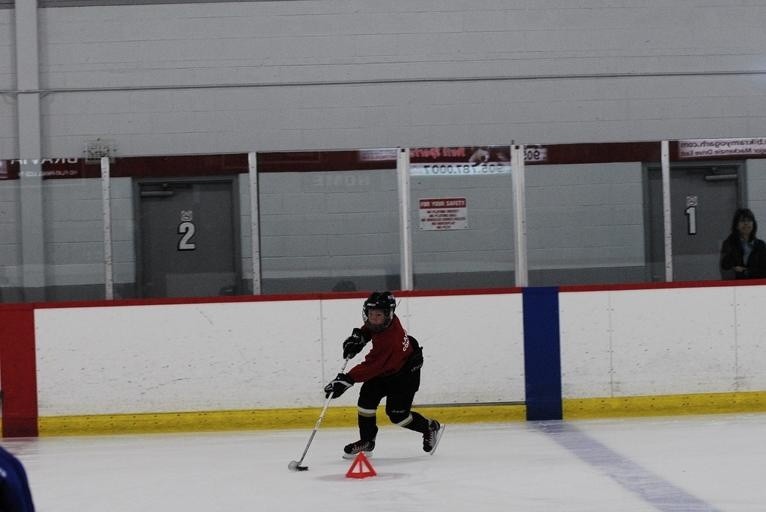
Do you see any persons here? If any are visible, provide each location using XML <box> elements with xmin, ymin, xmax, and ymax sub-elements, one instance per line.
<box><xmin>718</xmin><ymin>208</ymin><xmax>766</xmax><ymax>279</ymax></box>
<box><xmin>323</xmin><ymin>288</ymin><xmax>440</xmax><ymax>454</ymax></box>
<box><xmin>0</xmin><ymin>385</ymin><xmax>36</xmax><ymax>511</ymax></box>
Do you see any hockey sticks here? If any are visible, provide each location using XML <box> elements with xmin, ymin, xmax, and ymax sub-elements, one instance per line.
<box><xmin>289</xmin><ymin>355</ymin><xmax>351</xmax><ymax>470</ymax></box>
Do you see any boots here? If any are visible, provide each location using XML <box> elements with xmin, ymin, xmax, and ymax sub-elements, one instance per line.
<box><xmin>343</xmin><ymin>436</ymin><xmax>376</xmax><ymax>455</ymax></box>
<box><xmin>422</xmin><ymin>417</ymin><xmax>441</xmax><ymax>452</ymax></box>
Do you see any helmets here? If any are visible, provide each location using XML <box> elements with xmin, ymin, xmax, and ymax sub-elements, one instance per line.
<box><xmin>363</xmin><ymin>291</ymin><xmax>396</xmax><ymax>317</ymax></box>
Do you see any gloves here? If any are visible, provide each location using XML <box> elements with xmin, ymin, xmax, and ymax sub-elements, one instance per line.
<box><xmin>324</xmin><ymin>373</ymin><xmax>355</xmax><ymax>399</ymax></box>
<box><xmin>343</xmin><ymin>327</ymin><xmax>372</xmax><ymax>359</ymax></box>
